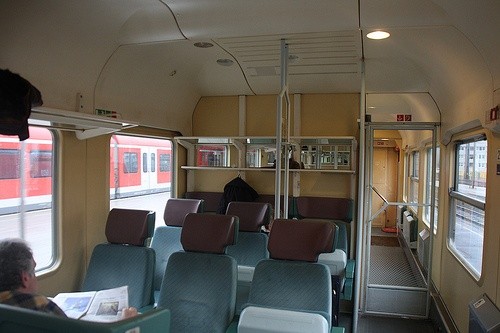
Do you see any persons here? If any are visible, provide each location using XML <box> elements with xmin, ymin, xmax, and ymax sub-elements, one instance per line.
<box><xmin>0</xmin><ymin>237</ymin><xmax>141</xmax><ymax>333</ymax></box>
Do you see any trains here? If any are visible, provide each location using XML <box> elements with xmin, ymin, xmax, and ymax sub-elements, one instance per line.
<box><xmin>0</xmin><ymin>125</ymin><xmax>225</xmax><ymax>214</ymax></box>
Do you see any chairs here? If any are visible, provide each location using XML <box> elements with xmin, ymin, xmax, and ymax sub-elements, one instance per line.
<box><xmin>226</xmin><ymin>218</ymin><xmax>345</xmax><ymax>333</ymax></box>
<box><xmin>148</xmin><ymin>198</ymin><xmax>205</xmax><ymax>307</ymax></box>
<box><xmin>80</xmin><ymin>208</ymin><xmax>157</xmax><ymax>314</ymax></box>
<box><xmin>294</xmin><ymin>197</ymin><xmax>355</xmax><ymax>325</ymax></box>
<box><xmin>223</xmin><ymin>202</ymin><xmax>272</xmax><ymax>317</ymax></box>
<box><xmin>156</xmin><ymin>212</ymin><xmax>239</xmax><ymax>333</ymax></box>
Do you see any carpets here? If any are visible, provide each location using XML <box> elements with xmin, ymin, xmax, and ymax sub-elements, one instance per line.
<box><xmin>381</xmin><ymin>227</ymin><xmax>400</xmax><ymax>233</ymax></box>
